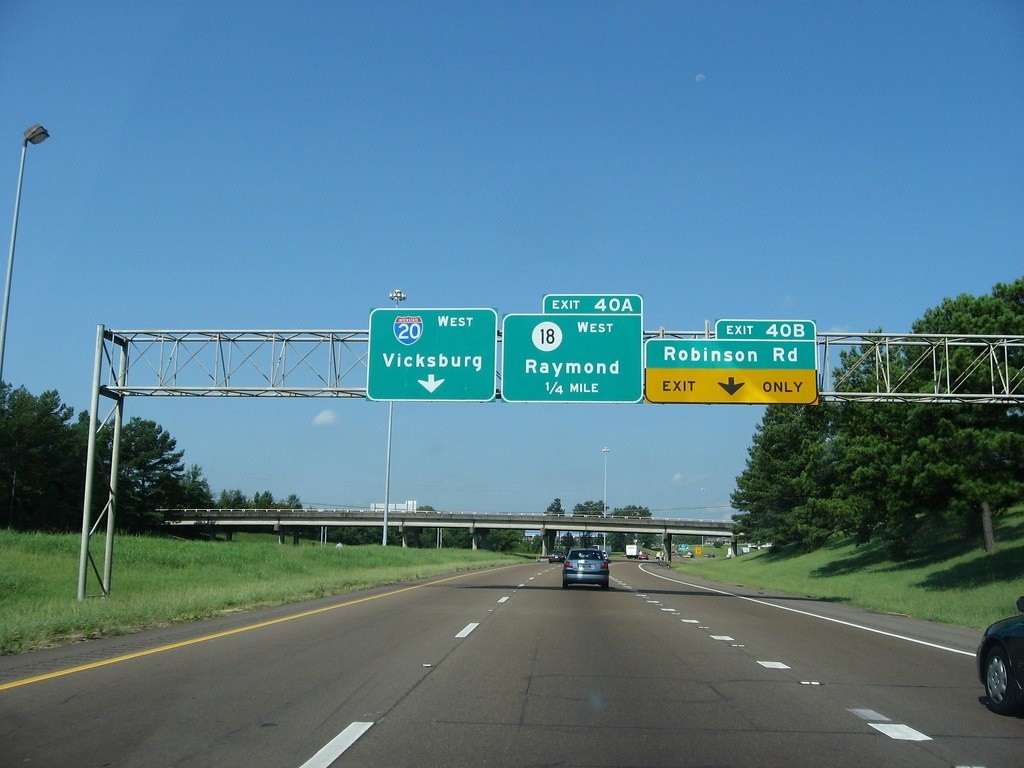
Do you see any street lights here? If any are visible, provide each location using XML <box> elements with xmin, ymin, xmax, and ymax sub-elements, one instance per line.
<box><xmin>1</xmin><ymin>123</ymin><xmax>50</xmax><ymax>391</ymax></box>
<box><xmin>602</xmin><ymin>446</ymin><xmax>610</xmax><ymax>520</ymax></box>
<box><xmin>381</xmin><ymin>288</ymin><xmax>408</xmax><ymax>546</ymax></box>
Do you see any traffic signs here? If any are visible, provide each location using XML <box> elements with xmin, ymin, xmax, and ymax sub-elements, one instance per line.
<box><xmin>368</xmin><ymin>308</ymin><xmax>497</xmax><ymax>401</ymax></box>
<box><xmin>640</xmin><ymin>318</ymin><xmax>819</xmax><ymax>406</ymax></box>
<box><xmin>502</xmin><ymin>293</ymin><xmax>642</xmax><ymax>405</ymax></box>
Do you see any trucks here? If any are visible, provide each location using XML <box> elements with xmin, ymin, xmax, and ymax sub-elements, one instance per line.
<box><xmin>626</xmin><ymin>545</ymin><xmax>640</xmax><ymax>559</ymax></box>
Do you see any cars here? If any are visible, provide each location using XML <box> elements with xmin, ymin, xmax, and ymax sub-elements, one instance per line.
<box><xmin>656</xmin><ymin>551</ymin><xmax>665</xmax><ymax>559</ymax></box>
<box><xmin>562</xmin><ymin>548</ymin><xmax>609</xmax><ymax>591</ymax></box>
<box><xmin>638</xmin><ymin>552</ymin><xmax>650</xmax><ymax>560</ymax></box>
<box><xmin>683</xmin><ymin>551</ymin><xmax>695</xmax><ymax>558</ymax></box>
<box><xmin>548</xmin><ymin>550</ymin><xmax>565</xmax><ymax>563</ymax></box>
<box><xmin>976</xmin><ymin>595</ymin><xmax>1024</xmax><ymax>715</ymax></box>
<box><xmin>705</xmin><ymin>552</ymin><xmax>715</xmax><ymax>558</ymax></box>
